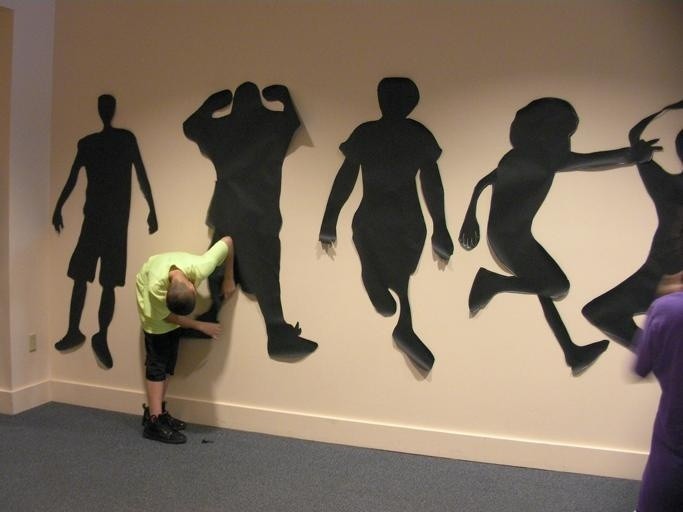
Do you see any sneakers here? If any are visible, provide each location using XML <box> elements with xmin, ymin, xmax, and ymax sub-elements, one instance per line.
<box><xmin>143</xmin><ymin>412</ymin><xmax>186</xmax><ymax>444</ymax></box>
<box><xmin>141</xmin><ymin>403</ymin><xmax>186</xmax><ymax>430</ymax></box>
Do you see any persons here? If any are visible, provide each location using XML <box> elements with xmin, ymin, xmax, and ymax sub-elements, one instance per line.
<box><xmin>624</xmin><ymin>237</ymin><xmax>682</xmax><ymax>512</ymax></box>
<box><xmin>134</xmin><ymin>235</ymin><xmax>237</xmax><ymax>444</ymax></box>
